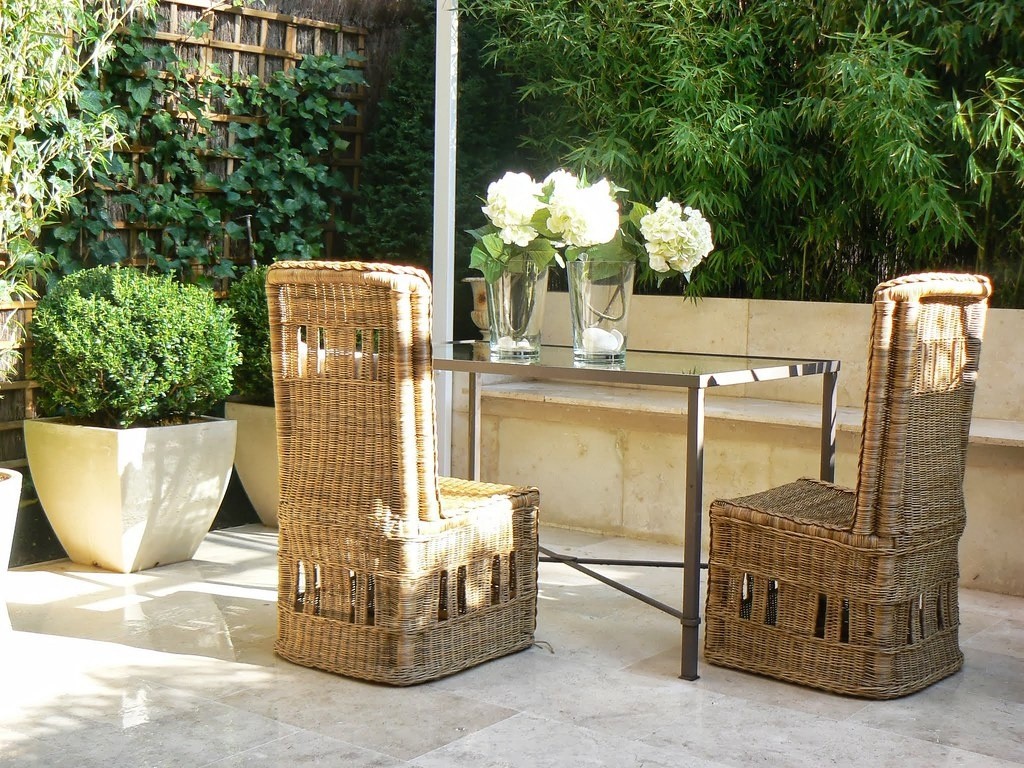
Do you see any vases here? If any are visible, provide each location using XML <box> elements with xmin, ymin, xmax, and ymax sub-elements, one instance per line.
<box><xmin>565</xmin><ymin>261</ymin><xmax>636</xmax><ymax>366</ymax></box>
<box><xmin>484</xmin><ymin>266</ymin><xmax>548</xmax><ymax>360</ymax></box>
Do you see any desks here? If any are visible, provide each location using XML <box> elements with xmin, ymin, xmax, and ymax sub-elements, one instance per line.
<box><xmin>431</xmin><ymin>339</ymin><xmax>841</xmax><ymax>682</ymax></box>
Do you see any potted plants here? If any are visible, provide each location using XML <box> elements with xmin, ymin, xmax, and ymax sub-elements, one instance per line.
<box><xmin>23</xmin><ymin>264</ymin><xmax>278</xmax><ymax>575</ymax></box>
<box><xmin>0</xmin><ymin>0</ymin><xmax>160</xmax><ymax>592</ymax></box>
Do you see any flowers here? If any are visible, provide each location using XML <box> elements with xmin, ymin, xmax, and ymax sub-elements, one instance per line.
<box><xmin>463</xmin><ymin>168</ymin><xmax>714</xmax><ymax>339</ymax></box>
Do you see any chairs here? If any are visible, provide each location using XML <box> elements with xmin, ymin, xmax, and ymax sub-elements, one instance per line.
<box><xmin>265</xmin><ymin>261</ymin><xmax>540</xmax><ymax>690</ymax></box>
<box><xmin>704</xmin><ymin>272</ymin><xmax>991</xmax><ymax>700</ymax></box>
